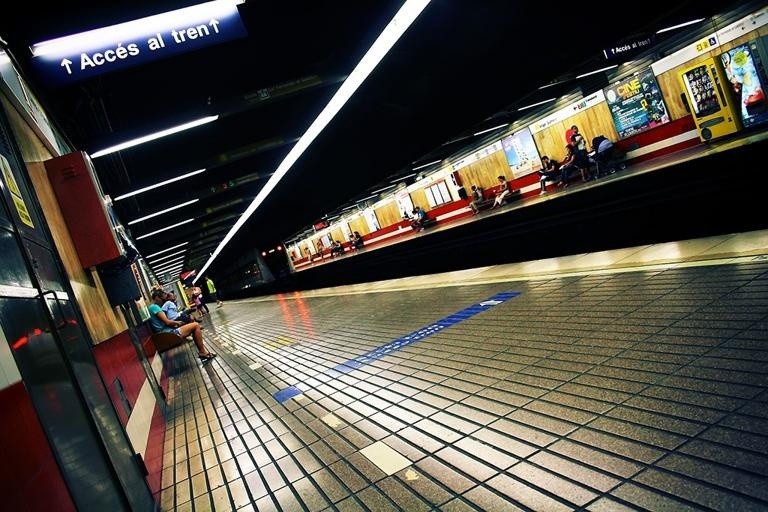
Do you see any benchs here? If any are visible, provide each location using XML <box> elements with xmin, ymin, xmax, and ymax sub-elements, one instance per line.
<box><xmin>151</xmin><ymin>331</ymin><xmax>194</xmax><ymax>353</ymax></box>
<box><xmin>553</xmin><ymin>165</ymin><xmax>596</xmax><ymax>187</ymax></box>
<box><xmin>409</xmin><ymin>217</ymin><xmax>437</xmax><ymax>230</ymax></box>
<box><xmin>465</xmin><ymin>190</ymin><xmax>520</xmax><ymax>210</ymax></box>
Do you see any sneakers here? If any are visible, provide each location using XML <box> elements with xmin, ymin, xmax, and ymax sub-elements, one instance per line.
<box><xmin>538</xmin><ymin>181</ymin><xmax>569</xmax><ymax>196</ymax></box>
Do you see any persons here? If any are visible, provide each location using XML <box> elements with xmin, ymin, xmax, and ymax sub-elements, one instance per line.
<box><xmin>329</xmin><ymin>240</ymin><xmax>344</xmax><ymax>259</ymax></box>
<box><xmin>159</xmin><ymin>290</ymin><xmax>209</xmax><ymax>330</ymax></box>
<box><xmin>149</xmin><ymin>287</ymin><xmax>217</xmax><ymax>364</ymax></box>
<box><xmin>205</xmin><ymin>276</ymin><xmax>223</xmax><ymax>308</ymax></box>
<box><xmin>316</xmin><ymin>242</ymin><xmax>322</xmax><ymax>258</ymax></box>
<box><xmin>469</xmin><ymin>185</ymin><xmax>483</xmax><ymax>215</ymax></box>
<box><xmin>404</xmin><ymin>207</ymin><xmax>425</xmax><ymax>231</ymax></box>
<box><xmin>490</xmin><ymin>176</ymin><xmax>510</xmax><ymax>209</ymax></box>
<box><xmin>304</xmin><ymin>247</ymin><xmax>314</xmax><ymax>266</ymax></box>
<box><xmin>539</xmin><ymin>125</ymin><xmax>589</xmax><ymax>196</ymax></box>
<box><xmin>348</xmin><ymin>231</ymin><xmax>363</xmax><ymax>249</ymax></box>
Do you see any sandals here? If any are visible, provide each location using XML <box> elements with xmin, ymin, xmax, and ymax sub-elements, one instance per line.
<box><xmin>198</xmin><ymin>351</ymin><xmax>217</xmax><ymax>364</ymax></box>
